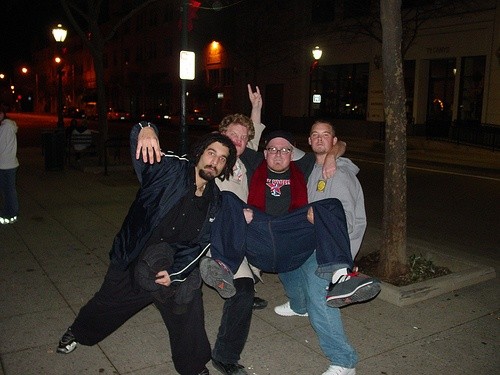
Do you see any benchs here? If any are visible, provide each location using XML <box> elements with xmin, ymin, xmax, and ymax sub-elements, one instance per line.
<box><xmin>40</xmin><ymin>129</ymin><xmax>67</xmax><ymax>159</ymax></box>
<box><xmin>58</xmin><ymin>130</ymin><xmax>121</xmax><ymax>169</ymax></box>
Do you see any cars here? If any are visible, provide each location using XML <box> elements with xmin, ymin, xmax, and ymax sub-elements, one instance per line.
<box><xmin>105</xmin><ymin>106</ymin><xmax>131</xmax><ymax>122</ymax></box>
<box><xmin>138</xmin><ymin>109</ymin><xmax>170</xmax><ymax>126</ymax></box>
<box><xmin>64</xmin><ymin>105</ymin><xmax>85</xmax><ymax>118</ymax></box>
<box><xmin>177</xmin><ymin>113</ymin><xmax>212</xmax><ymax>131</ymax></box>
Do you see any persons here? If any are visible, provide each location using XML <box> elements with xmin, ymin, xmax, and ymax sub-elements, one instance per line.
<box><xmin>64</xmin><ymin>120</ymin><xmax>78</xmax><ymax>136</ymax></box>
<box><xmin>0</xmin><ymin>108</ymin><xmax>19</xmax><ymax>223</ymax></box>
<box><xmin>273</xmin><ymin>116</ymin><xmax>367</xmax><ymax>375</ymax></box>
<box><xmin>57</xmin><ymin>120</ymin><xmax>237</xmax><ymax>375</ymax></box>
<box><xmin>71</xmin><ymin>120</ymin><xmax>96</xmax><ymax>163</ymax></box>
<box><xmin>201</xmin><ymin>141</ymin><xmax>381</xmax><ymax>309</ymax></box>
<box><xmin>211</xmin><ymin>84</ymin><xmax>266</xmax><ymax>375</ymax></box>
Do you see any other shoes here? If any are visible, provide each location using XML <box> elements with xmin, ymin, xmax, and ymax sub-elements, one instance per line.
<box><xmin>0</xmin><ymin>216</ymin><xmax>17</xmax><ymax>224</ymax></box>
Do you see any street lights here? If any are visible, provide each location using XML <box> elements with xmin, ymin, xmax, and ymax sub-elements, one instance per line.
<box><xmin>51</xmin><ymin>22</ymin><xmax>68</xmax><ymax>173</ymax></box>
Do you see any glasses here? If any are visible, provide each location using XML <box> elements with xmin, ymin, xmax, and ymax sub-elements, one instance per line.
<box><xmin>268</xmin><ymin>146</ymin><xmax>292</xmax><ymax>155</ymax></box>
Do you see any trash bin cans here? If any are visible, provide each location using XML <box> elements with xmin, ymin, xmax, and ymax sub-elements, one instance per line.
<box><xmin>39</xmin><ymin>128</ymin><xmax>66</xmax><ymax>171</ymax></box>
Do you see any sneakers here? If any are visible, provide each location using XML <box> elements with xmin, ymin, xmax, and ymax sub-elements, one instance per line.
<box><xmin>253</xmin><ymin>297</ymin><xmax>268</xmax><ymax>311</ymax></box>
<box><xmin>198</xmin><ymin>368</ymin><xmax>210</xmax><ymax>375</ymax></box>
<box><xmin>325</xmin><ymin>272</ymin><xmax>381</xmax><ymax>307</ymax></box>
<box><xmin>205</xmin><ymin>358</ymin><xmax>249</xmax><ymax>375</ymax></box>
<box><xmin>321</xmin><ymin>365</ymin><xmax>356</xmax><ymax>375</ymax></box>
<box><xmin>56</xmin><ymin>327</ymin><xmax>78</xmax><ymax>353</ymax></box>
<box><xmin>274</xmin><ymin>301</ymin><xmax>309</xmax><ymax>316</ymax></box>
<box><xmin>199</xmin><ymin>256</ymin><xmax>236</xmax><ymax>298</ymax></box>
